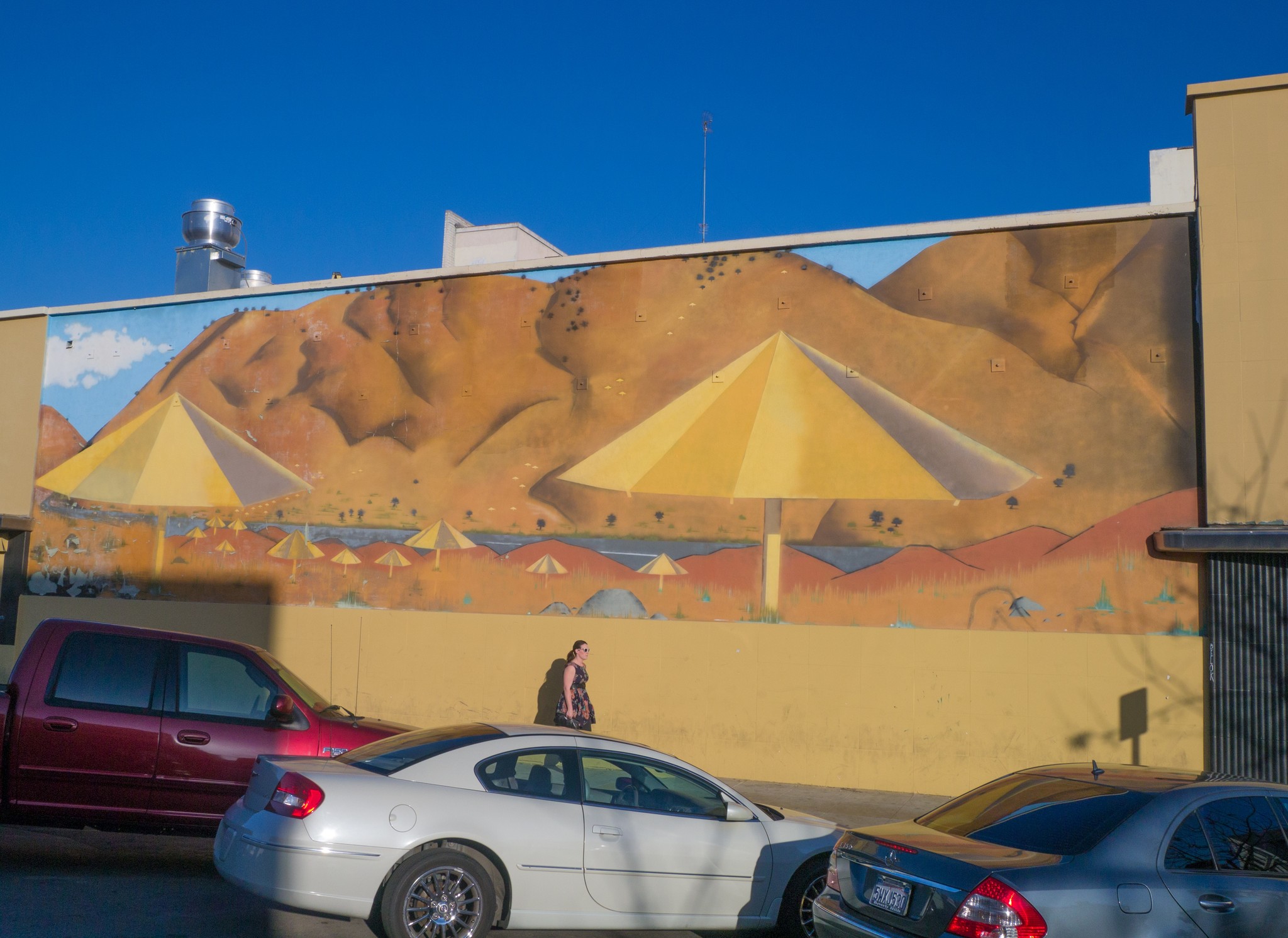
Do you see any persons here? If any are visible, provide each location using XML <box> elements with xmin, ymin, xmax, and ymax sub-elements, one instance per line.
<box><xmin>555</xmin><ymin>640</ymin><xmax>596</xmax><ymax>732</ymax></box>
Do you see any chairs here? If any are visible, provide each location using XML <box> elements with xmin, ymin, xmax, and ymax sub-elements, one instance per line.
<box><xmin>528</xmin><ymin>765</ymin><xmax>591</xmax><ymax>801</ymax></box>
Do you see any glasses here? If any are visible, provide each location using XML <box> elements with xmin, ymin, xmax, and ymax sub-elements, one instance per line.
<box><xmin>577</xmin><ymin>648</ymin><xmax>590</xmax><ymax>653</ymax></box>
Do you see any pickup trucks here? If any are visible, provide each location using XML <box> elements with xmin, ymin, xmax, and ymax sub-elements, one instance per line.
<box><xmin>1</xmin><ymin>616</ymin><xmax>431</xmax><ymax>838</ymax></box>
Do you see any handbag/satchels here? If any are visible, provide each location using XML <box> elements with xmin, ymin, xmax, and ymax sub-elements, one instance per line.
<box><xmin>556</xmin><ymin>716</ymin><xmax>578</xmax><ymax>729</ymax></box>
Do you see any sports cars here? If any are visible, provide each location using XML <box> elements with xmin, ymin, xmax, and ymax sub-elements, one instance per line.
<box><xmin>212</xmin><ymin>721</ymin><xmax>849</xmax><ymax>938</ymax></box>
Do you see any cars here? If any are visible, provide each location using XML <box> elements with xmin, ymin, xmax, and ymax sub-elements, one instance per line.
<box><xmin>808</xmin><ymin>760</ymin><xmax>1288</xmax><ymax>938</ymax></box>
